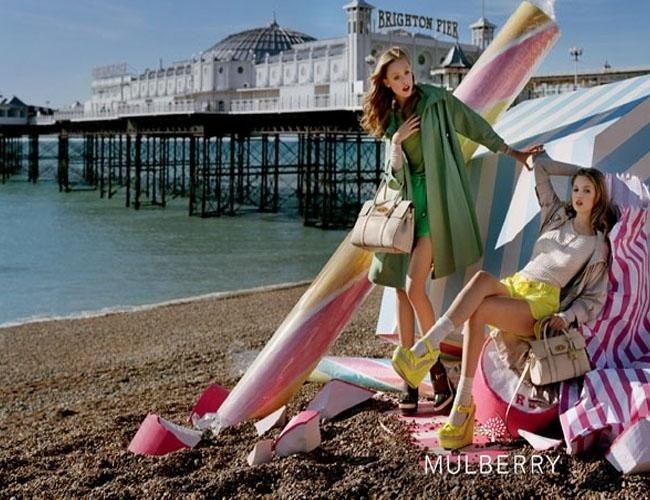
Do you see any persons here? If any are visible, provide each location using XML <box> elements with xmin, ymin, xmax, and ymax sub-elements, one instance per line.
<box><xmin>359</xmin><ymin>47</ymin><xmax>545</xmax><ymax>417</ymax></box>
<box><xmin>391</xmin><ymin>152</ymin><xmax>610</xmax><ymax>450</ymax></box>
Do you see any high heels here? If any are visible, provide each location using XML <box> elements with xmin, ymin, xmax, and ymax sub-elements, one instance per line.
<box><xmin>391</xmin><ymin>336</ymin><xmax>440</xmax><ymax>388</ymax></box>
<box><xmin>439</xmin><ymin>393</ymin><xmax>478</xmax><ymax>451</ymax></box>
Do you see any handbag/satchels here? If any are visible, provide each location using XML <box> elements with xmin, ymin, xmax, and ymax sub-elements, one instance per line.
<box><xmin>351</xmin><ymin>197</ymin><xmax>412</xmax><ymax>256</ymax></box>
<box><xmin>530</xmin><ymin>330</ymin><xmax>590</xmax><ymax>385</ymax></box>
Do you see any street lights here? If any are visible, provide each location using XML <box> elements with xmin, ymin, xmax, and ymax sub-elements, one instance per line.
<box><xmin>602</xmin><ymin>57</ymin><xmax>613</xmax><ymax>74</ymax></box>
<box><xmin>569</xmin><ymin>45</ymin><xmax>585</xmax><ymax>95</ymax></box>
<box><xmin>364</xmin><ymin>53</ymin><xmax>376</xmax><ymax>76</ymax></box>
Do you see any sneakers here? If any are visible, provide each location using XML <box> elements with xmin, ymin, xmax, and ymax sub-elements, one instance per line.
<box><xmin>398</xmin><ymin>394</ymin><xmax>417</xmax><ymax>413</ymax></box>
<box><xmin>432</xmin><ymin>384</ymin><xmax>455</xmax><ymax>412</ymax></box>
